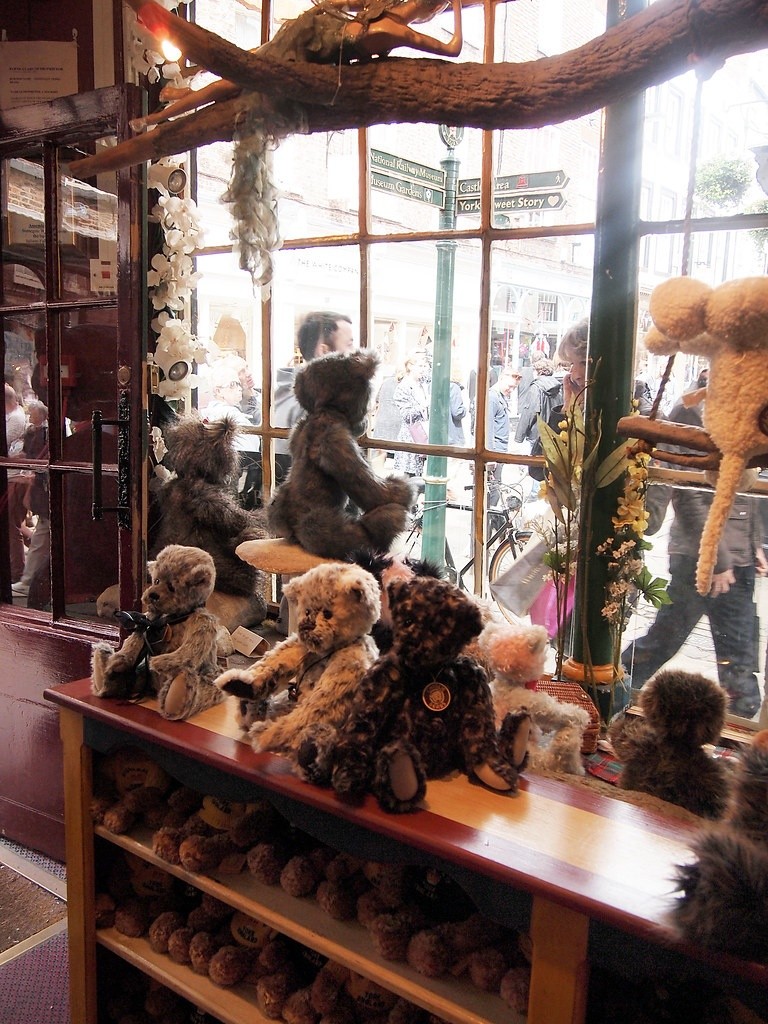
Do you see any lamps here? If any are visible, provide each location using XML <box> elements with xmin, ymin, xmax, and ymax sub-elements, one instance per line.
<box><xmin>149</xmin><ymin>162</ymin><xmax>187</xmax><ymax>194</ymax></box>
<box><xmin>153</xmin><ymin>349</ymin><xmax>189</xmax><ymax>383</ymax></box>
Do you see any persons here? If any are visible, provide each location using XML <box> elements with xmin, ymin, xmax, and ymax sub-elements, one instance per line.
<box><xmin>0</xmin><ymin>322</ymin><xmax>119</xmax><ymax>596</ymax></box>
<box><xmin>197</xmin><ymin>311</ymin><xmax>768</xmax><ymax>722</ymax></box>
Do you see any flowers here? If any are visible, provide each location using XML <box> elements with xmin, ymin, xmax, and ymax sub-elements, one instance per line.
<box><xmin>131</xmin><ymin>0</ymin><xmax>193</xmax><ymax>88</ymax></box>
<box><xmin>530</xmin><ymin>355</ymin><xmax>673</xmax><ymax>725</ymax></box>
<box><xmin>144</xmin><ymin>150</ymin><xmax>209</xmax><ymax>493</ymax></box>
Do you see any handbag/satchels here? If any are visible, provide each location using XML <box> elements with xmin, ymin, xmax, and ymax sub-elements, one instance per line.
<box><xmin>409</xmin><ymin>415</ymin><xmax>429</xmax><ymax>458</ymax></box>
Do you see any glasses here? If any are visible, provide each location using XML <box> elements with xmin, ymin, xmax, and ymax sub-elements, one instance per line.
<box><xmin>216</xmin><ymin>381</ymin><xmax>241</xmax><ymax>389</ymax></box>
<box><xmin>505</xmin><ymin>374</ymin><xmax>522</xmax><ymax>380</ymax></box>
<box><xmin>415</xmin><ymin>365</ymin><xmax>426</xmax><ymax>367</ymax></box>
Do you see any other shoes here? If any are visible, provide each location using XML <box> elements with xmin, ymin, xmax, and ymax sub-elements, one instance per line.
<box><xmin>720</xmin><ymin>737</ymin><xmax>750</xmax><ymax>750</ymax></box>
<box><xmin>524</xmin><ymin>496</ymin><xmax>539</xmax><ymax>503</ymax></box>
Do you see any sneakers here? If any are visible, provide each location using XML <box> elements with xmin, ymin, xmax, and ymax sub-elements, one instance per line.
<box><xmin>12</xmin><ymin>582</ymin><xmax>29</xmax><ymax>595</ymax></box>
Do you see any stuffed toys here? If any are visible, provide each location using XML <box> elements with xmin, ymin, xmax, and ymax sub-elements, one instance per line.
<box><xmin>149</xmin><ymin>408</ymin><xmax>279</xmax><ymax>595</ymax></box>
<box><xmin>269</xmin><ymin>351</ymin><xmax>413</xmax><ymax>559</ymax></box>
<box><xmin>295</xmin><ymin>575</ymin><xmax>519</xmax><ymax>814</ymax></box>
<box><xmin>606</xmin><ymin>670</ymin><xmax>736</xmax><ymax>823</ymax></box>
<box><xmin>642</xmin><ymin>275</ymin><xmax>768</xmax><ymax>597</ymax></box>
<box><xmin>460</xmin><ymin>619</ymin><xmax>590</xmax><ymax>778</ymax></box>
<box><xmin>92</xmin><ymin>541</ymin><xmax>228</xmax><ymax>721</ymax></box>
<box><xmin>212</xmin><ymin>563</ymin><xmax>380</xmax><ymax>776</ymax></box>
<box><xmin>86</xmin><ymin>726</ymin><xmax>768</xmax><ymax>1024</ymax></box>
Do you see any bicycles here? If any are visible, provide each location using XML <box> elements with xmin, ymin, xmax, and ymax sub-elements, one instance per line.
<box><xmin>389</xmin><ymin>460</ymin><xmax>567</xmax><ymax>634</ymax></box>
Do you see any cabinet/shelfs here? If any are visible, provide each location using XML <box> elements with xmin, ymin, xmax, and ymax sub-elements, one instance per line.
<box><xmin>44</xmin><ymin>678</ymin><xmax>767</xmax><ymax>1024</ymax></box>
